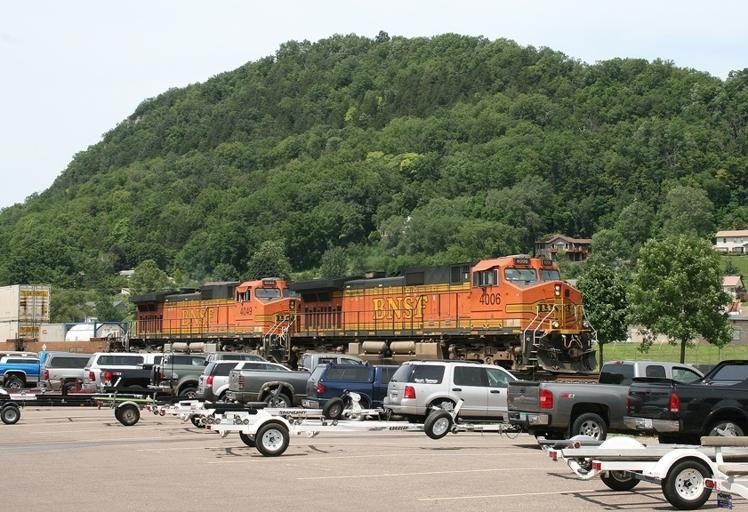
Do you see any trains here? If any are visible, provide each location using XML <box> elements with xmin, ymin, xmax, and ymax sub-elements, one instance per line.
<box><xmin>341</xmin><ymin>250</ymin><xmax>600</xmax><ymax>382</ymax></box>
<box><xmin>124</xmin><ymin>275</ymin><xmax>304</xmax><ymax>356</ymax></box>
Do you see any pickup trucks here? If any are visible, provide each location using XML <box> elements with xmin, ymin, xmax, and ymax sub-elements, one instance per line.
<box><xmin>624</xmin><ymin>359</ymin><xmax>747</xmax><ymax>444</ymax></box>
<box><xmin>303</xmin><ymin>363</ymin><xmax>401</xmax><ymax>420</ymax></box>
<box><xmin>504</xmin><ymin>360</ymin><xmax>707</xmax><ymax>442</ymax></box>
<box><xmin>0</xmin><ymin>346</ymin><xmax>293</xmax><ymax>405</ymax></box>
<box><xmin>228</xmin><ymin>352</ymin><xmax>370</xmax><ymax>409</ymax></box>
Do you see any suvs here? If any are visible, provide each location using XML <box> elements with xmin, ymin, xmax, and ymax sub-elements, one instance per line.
<box><xmin>382</xmin><ymin>359</ymin><xmax>524</xmax><ymax>430</ymax></box>
